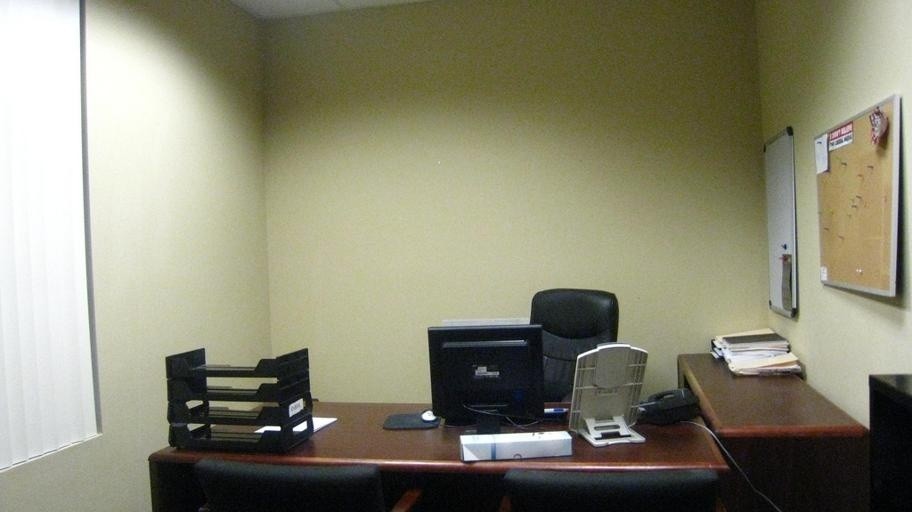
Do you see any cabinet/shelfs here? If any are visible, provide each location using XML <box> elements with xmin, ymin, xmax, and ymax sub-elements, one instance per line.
<box><xmin>162</xmin><ymin>348</ymin><xmax>314</xmax><ymax>454</ymax></box>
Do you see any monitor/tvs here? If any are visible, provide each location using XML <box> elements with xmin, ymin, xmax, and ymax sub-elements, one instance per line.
<box><xmin>428</xmin><ymin>324</ymin><xmax>544</xmax><ymax>434</ymax></box>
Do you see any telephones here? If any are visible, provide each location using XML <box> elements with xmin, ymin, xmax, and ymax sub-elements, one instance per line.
<box><xmin>640</xmin><ymin>388</ymin><xmax>703</xmax><ymax>427</ymax></box>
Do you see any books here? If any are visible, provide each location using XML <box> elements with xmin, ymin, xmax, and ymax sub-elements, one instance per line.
<box><xmin>713</xmin><ymin>327</ymin><xmax>803</xmax><ymax>376</ymax></box>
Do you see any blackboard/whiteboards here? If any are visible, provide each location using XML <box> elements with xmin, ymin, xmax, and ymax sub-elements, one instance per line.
<box><xmin>763</xmin><ymin>126</ymin><xmax>799</xmax><ymax>318</ymax></box>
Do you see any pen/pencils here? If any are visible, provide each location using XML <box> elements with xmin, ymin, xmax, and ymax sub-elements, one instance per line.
<box><xmin>543</xmin><ymin>408</ymin><xmax>570</xmax><ymax>414</ymax></box>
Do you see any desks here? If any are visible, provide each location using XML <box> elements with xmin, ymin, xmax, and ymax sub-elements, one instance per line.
<box><xmin>145</xmin><ymin>400</ymin><xmax>732</xmax><ymax>511</ymax></box>
<box><xmin>674</xmin><ymin>351</ymin><xmax>874</xmax><ymax>511</ymax></box>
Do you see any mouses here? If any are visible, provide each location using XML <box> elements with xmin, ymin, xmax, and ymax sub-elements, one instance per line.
<box><xmin>421</xmin><ymin>409</ymin><xmax>437</xmax><ymax>421</ymax></box>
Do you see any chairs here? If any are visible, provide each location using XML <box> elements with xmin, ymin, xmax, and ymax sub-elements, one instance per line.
<box><xmin>525</xmin><ymin>287</ymin><xmax>620</xmax><ymax>403</ymax></box>
